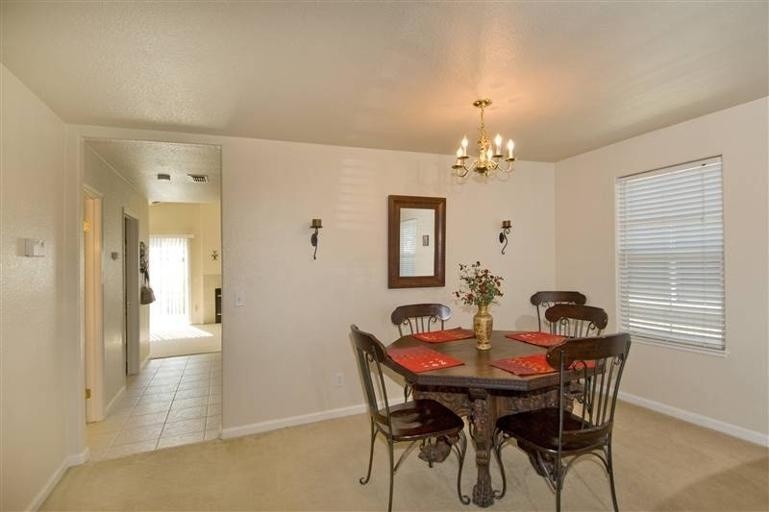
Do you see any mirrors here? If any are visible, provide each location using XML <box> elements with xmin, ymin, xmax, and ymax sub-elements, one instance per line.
<box><xmin>388</xmin><ymin>196</ymin><xmax>446</xmax><ymax>287</ymax></box>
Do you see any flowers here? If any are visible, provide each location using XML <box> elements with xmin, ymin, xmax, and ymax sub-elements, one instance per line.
<box><xmin>452</xmin><ymin>257</ymin><xmax>506</xmax><ymax>306</ymax></box>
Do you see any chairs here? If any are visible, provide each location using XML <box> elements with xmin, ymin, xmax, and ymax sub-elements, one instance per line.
<box><xmin>345</xmin><ymin>323</ymin><xmax>473</xmax><ymax>511</ymax></box>
<box><xmin>544</xmin><ymin>304</ymin><xmax>609</xmax><ymax>337</ymax></box>
<box><xmin>489</xmin><ymin>333</ymin><xmax>633</xmax><ymax>512</ymax></box>
<box><xmin>529</xmin><ymin>291</ymin><xmax>586</xmax><ymax>332</ymax></box>
<box><xmin>390</xmin><ymin>302</ymin><xmax>453</xmax><ymax>335</ymax></box>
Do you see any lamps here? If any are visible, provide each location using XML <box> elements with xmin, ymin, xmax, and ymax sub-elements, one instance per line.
<box><xmin>499</xmin><ymin>219</ymin><xmax>513</xmax><ymax>256</ymax></box>
<box><xmin>451</xmin><ymin>98</ymin><xmax>517</xmax><ymax>183</ymax></box>
<box><xmin>308</xmin><ymin>216</ymin><xmax>324</xmax><ymax>262</ymax></box>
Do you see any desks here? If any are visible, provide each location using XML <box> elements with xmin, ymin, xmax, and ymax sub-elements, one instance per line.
<box><xmin>385</xmin><ymin>327</ymin><xmax>608</xmax><ymax>505</ymax></box>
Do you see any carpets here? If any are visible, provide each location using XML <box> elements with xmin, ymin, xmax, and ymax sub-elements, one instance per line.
<box><xmin>149</xmin><ymin>323</ymin><xmax>221</xmax><ymax>360</ymax></box>
